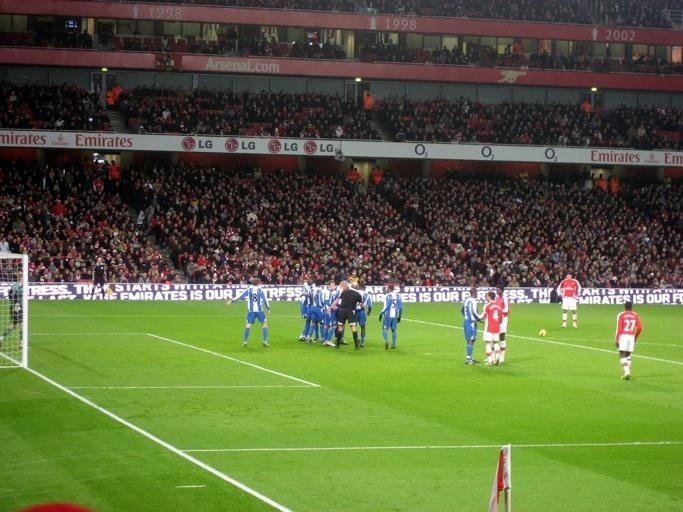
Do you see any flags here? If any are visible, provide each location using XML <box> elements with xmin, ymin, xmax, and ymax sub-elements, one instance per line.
<box><xmin>487</xmin><ymin>442</ymin><xmax>512</xmax><ymax>511</ymax></box>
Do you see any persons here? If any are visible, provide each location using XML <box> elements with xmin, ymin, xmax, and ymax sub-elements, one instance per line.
<box><xmin>206</xmin><ymin>1</ymin><xmax>683</xmax><ymax>30</ymax></box>
<box><xmin>495</xmin><ymin>286</ymin><xmax>509</xmax><ymax>362</ymax></box>
<box><xmin>556</xmin><ymin>272</ymin><xmax>581</xmax><ymax>328</ymax></box>
<box><xmin>221</xmin><ymin>278</ymin><xmax>271</xmax><ymax>347</ymax></box>
<box><xmin>479</xmin><ymin>292</ymin><xmax>502</xmax><ymax>366</ymax></box>
<box><xmin>0</xmin><ymin>273</ymin><xmax>28</xmax><ymax>347</ymax></box>
<box><xmin>460</xmin><ymin>287</ymin><xmax>482</xmax><ymax>365</ymax></box>
<box><xmin>614</xmin><ymin>300</ymin><xmax>642</xmax><ymax>381</ymax></box>
<box><xmin>300</xmin><ymin>275</ymin><xmax>372</xmax><ymax>350</ymax></box>
<box><xmin>89</xmin><ymin>258</ymin><xmax>106</xmax><ymax>301</ymax></box>
<box><xmin>1</xmin><ymin>79</ymin><xmax>683</xmax><ymax>152</ymax></box>
<box><xmin>2</xmin><ymin>157</ymin><xmax>683</xmax><ymax>289</ymax></box>
<box><xmin>378</xmin><ymin>285</ymin><xmax>401</xmax><ymax>350</ymax></box>
<box><xmin>2</xmin><ymin>28</ymin><xmax>681</xmax><ymax>76</ymax></box>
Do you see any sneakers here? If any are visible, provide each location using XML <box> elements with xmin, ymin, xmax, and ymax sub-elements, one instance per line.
<box><xmin>19</xmin><ymin>341</ymin><xmax>31</xmax><ymax>350</ymax></box>
<box><xmin>573</xmin><ymin>324</ymin><xmax>578</xmax><ymax>329</ymax></box>
<box><xmin>620</xmin><ymin>371</ymin><xmax>632</xmax><ymax>380</ymax></box>
<box><xmin>0</xmin><ymin>335</ymin><xmax>5</xmax><ymax>342</ymax></box>
<box><xmin>298</xmin><ymin>335</ymin><xmax>365</xmax><ymax>351</ymax></box>
<box><xmin>464</xmin><ymin>358</ymin><xmax>506</xmax><ymax>367</ymax></box>
<box><xmin>262</xmin><ymin>341</ymin><xmax>270</xmax><ymax>347</ymax></box>
<box><xmin>561</xmin><ymin>324</ymin><xmax>567</xmax><ymax>328</ymax></box>
<box><xmin>385</xmin><ymin>342</ymin><xmax>389</xmax><ymax>351</ymax></box>
<box><xmin>243</xmin><ymin>341</ymin><xmax>248</xmax><ymax>348</ymax></box>
<box><xmin>390</xmin><ymin>346</ymin><xmax>397</xmax><ymax>349</ymax></box>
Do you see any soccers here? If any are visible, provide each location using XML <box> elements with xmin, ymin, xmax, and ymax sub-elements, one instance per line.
<box><xmin>539</xmin><ymin>329</ymin><xmax>546</xmax><ymax>337</ymax></box>
